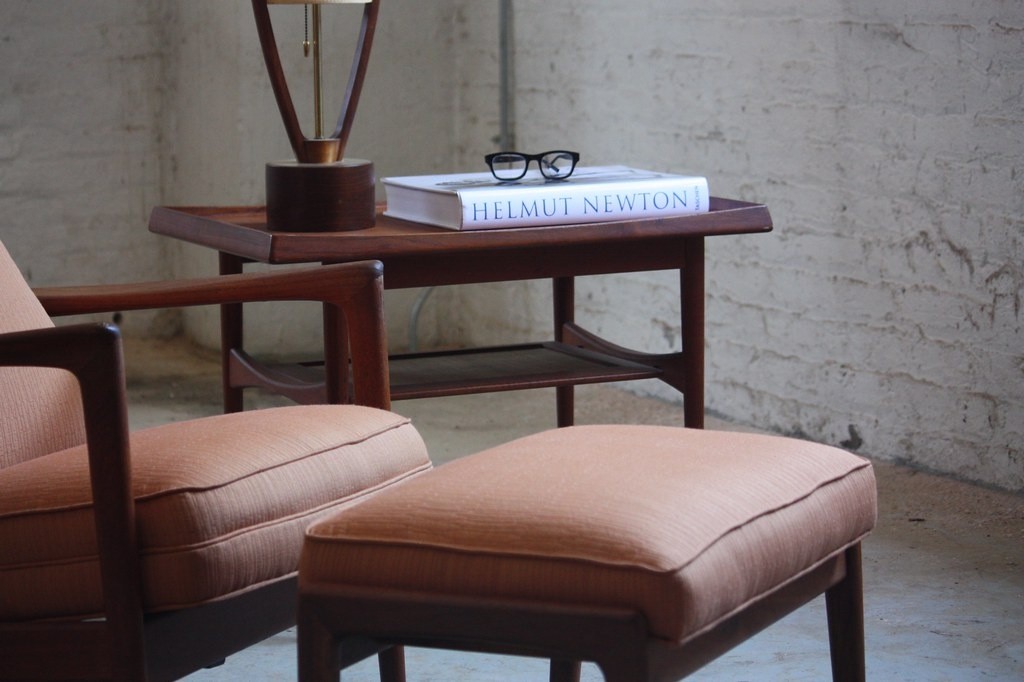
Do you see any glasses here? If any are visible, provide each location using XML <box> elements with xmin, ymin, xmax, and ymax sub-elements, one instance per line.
<box><xmin>485</xmin><ymin>150</ymin><xmax>580</xmax><ymax>181</ymax></box>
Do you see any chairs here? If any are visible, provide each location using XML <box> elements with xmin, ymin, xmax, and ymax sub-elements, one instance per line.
<box><xmin>0</xmin><ymin>239</ymin><xmax>435</xmax><ymax>682</ymax></box>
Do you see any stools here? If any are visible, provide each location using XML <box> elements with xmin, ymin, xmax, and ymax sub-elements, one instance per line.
<box><xmin>298</xmin><ymin>423</ymin><xmax>878</xmax><ymax>682</ymax></box>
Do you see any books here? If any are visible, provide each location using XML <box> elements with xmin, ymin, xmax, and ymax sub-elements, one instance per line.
<box><xmin>380</xmin><ymin>165</ymin><xmax>710</xmax><ymax>231</ymax></box>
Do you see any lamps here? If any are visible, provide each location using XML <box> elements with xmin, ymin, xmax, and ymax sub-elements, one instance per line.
<box><xmin>252</xmin><ymin>0</ymin><xmax>381</xmax><ymax>233</ymax></box>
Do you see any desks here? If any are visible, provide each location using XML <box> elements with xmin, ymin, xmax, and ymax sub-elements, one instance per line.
<box><xmin>147</xmin><ymin>196</ymin><xmax>772</xmax><ymax>430</ymax></box>
